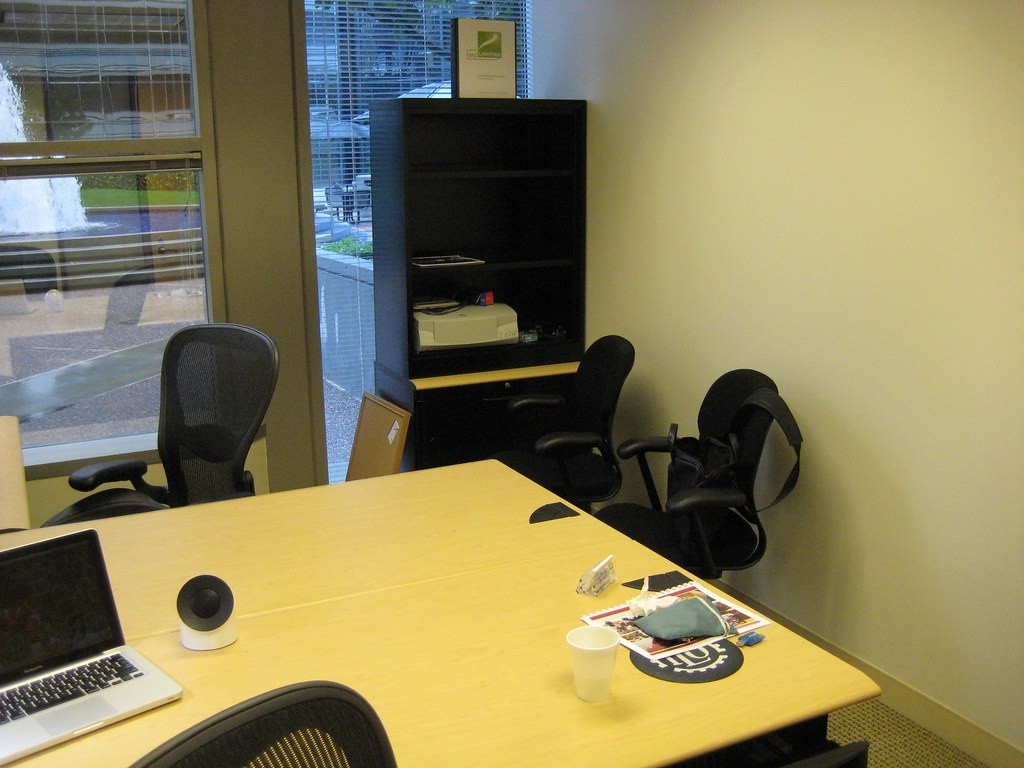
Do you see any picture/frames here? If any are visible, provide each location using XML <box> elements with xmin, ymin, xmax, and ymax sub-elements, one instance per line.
<box><xmin>345</xmin><ymin>390</ymin><xmax>413</xmax><ymax>482</ymax></box>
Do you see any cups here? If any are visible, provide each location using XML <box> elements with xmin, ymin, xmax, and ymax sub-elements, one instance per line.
<box><xmin>566</xmin><ymin>626</ymin><xmax>620</xmax><ymax>701</ymax></box>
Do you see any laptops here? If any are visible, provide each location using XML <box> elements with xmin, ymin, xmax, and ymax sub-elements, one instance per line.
<box><xmin>0</xmin><ymin>527</ymin><xmax>183</xmax><ymax>767</ymax></box>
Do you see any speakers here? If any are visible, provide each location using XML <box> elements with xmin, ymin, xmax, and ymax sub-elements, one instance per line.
<box><xmin>176</xmin><ymin>574</ymin><xmax>237</xmax><ymax>651</ymax></box>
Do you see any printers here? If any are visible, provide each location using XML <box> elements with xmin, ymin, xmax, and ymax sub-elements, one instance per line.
<box><xmin>409</xmin><ymin>302</ymin><xmax>520</xmax><ymax>352</ymax></box>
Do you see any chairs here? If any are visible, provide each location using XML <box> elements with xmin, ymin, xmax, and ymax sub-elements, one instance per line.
<box><xmin>490</xmin><ymin>334</ymin><xmax>635</xmax><ymax>511</ymax></box>
<box><xmin>41</xmin><ymin>322</ymin><xmax>281</xmax><ymax>527</ymax></box>
<box><xmin>130</xmin><ymin>680</ymin><xmax>398</xmax><ymax>768</ymax></box>
<box><xmin>594</xmin><ymin>368</ymin><xmax>779</xmax><ymax>585</ymax></box>
<box><xmin>101</xmin><ymin>265</ymin><xmax>155</xmax><ymax>337</ymax></box>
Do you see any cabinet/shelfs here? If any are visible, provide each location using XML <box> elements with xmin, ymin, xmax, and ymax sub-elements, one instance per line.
<box><xmin>367</xmin><ymin>96</ymin><xmax>589</xmax><ymax>470</ymax></box>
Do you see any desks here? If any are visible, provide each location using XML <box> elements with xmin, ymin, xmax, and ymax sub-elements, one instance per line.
<box><xmin>0</xmin><ymin>414</ymin><xmax>883</xmax><ymax>768</ymax></box>
